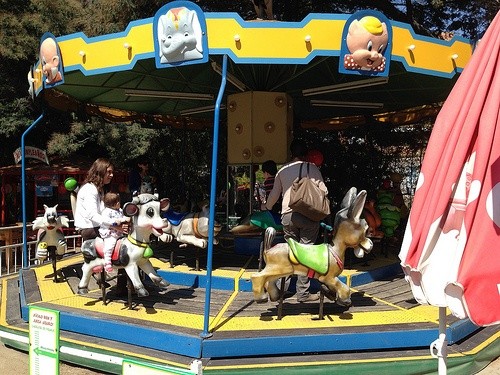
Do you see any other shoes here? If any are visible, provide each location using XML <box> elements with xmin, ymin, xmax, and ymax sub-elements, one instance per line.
<box><xmin>104</xmin><ymin>261</ymin><xmax>113</xmax><ymax>273</ymax></box>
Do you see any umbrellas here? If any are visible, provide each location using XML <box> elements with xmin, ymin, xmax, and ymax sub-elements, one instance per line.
<box><xmin>398</xmin><ymin>10</ymin><xmax>500</xmax><ymax>375</ymax></box>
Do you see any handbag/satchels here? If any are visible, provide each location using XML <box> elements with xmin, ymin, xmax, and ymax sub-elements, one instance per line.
<box><xmin>288</xmin><ymin>175</ymin><xmax>331</xmax><ymax>222</ymax></box>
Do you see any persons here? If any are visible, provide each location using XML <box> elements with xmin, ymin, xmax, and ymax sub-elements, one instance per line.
<box><xmin>74</xmin><ymin>158</ymin><xmax>131</xmax><ymax>240</ymax></box>
<box><xmin>98</xmin><ymin>192</ymin><xmax>125</xmax><ymax>273</ymax></box>
<box><xmin>260</xmin><ymin>140</ymin><xmax>328</xmax><ymax>301</ymax></box>
<box><xmin>166</xmin><ymin>173</ymin><xmax>186</xmax><ymax>203</ymax></box>
<box><xmin>262</xmin><ymin>160</ymin><xmax>282</xmax><ymax>212</ymax></box>
<box><xmin>252</xmin><ymin>165</ymin><xmax>259</xmax><ymax>187</ymax></box>
<box><xmin>217</xmin><ymin>163</ymin><xmax>235</xmax><ymax>197</ymax></box>
